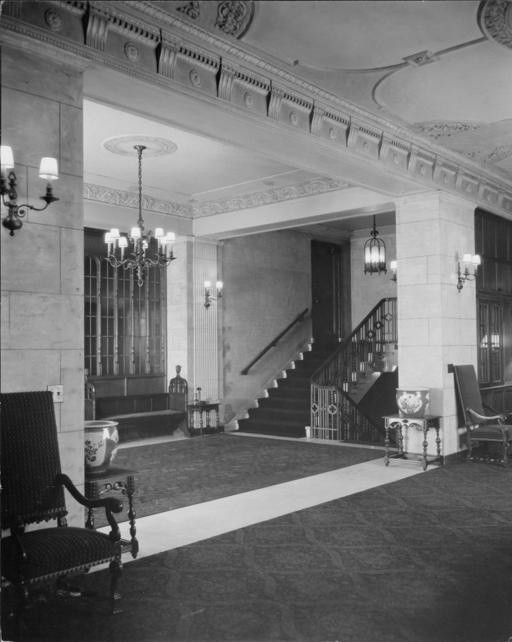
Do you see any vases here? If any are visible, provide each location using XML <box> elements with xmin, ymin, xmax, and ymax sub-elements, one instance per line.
<box><xmin>84</xmin><ymin>420</ymin><xmax>119</xmax><ymax>475</ymax></box>
<box><xmin>396</xmin><ymin>387</ymin><xmax>429</xmax><ymax>419</ymax></box>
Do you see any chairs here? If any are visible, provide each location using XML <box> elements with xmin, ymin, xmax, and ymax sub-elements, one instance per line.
<box><xmin>454</xmin><ymin>365</ymin><xmax>512</xmax><ymax>465</ymax></box>
<box><xmin>0</xmin><ymin>391</ymin><xmax>121</xmax><ymax>642</ymax></box>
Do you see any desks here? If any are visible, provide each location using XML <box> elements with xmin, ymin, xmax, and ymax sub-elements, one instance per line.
<box><xmin>84</xmin><ymin>469</ymin><xmax>141</xmax><ymax>559</ymax></box>
<box><xmin>381</xmin><ymin>414</ymin><xmax>442</xmax><ymax>471</ymax></box>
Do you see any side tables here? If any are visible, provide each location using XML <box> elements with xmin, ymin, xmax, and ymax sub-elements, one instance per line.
<box><xmin>189</xmin><ymin>404</ymin><xmax>220</xmax><ymax>435</ymax></box>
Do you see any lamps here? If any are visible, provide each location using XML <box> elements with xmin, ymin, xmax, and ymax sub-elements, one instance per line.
<box><xmin>205</xmin><ymin>281</ymin><xmax>223</xmax><ymax>307</ymax></box>
<box><xmin>105</xmin><ymin>145</ymin><xmax>175</xmax><ymax>288</ymax></box>
<box><xmin>0</xmin><ymin>146</ymin><xmax>60</xmax><ymax>236</ymax></box>
<box><xmin>364</xmin><ymin>215</ymin><xmax>387</xmax><ymax>275</ymax></box>
<box><xmin>457</xmin><ymin>254</ymin><xmax>481</xmax><ymax>292</ymax></box>
<box><xmin>389</xmin><ymin>261</ymin><xmax>396</xmax><ymax>281</ymax></box>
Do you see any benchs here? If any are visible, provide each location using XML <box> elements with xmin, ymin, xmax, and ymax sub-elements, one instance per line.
<box><xmin>84</xmin><ymin>392</ymin><xmax>188</xmax><ymax>442</ymax></box>
